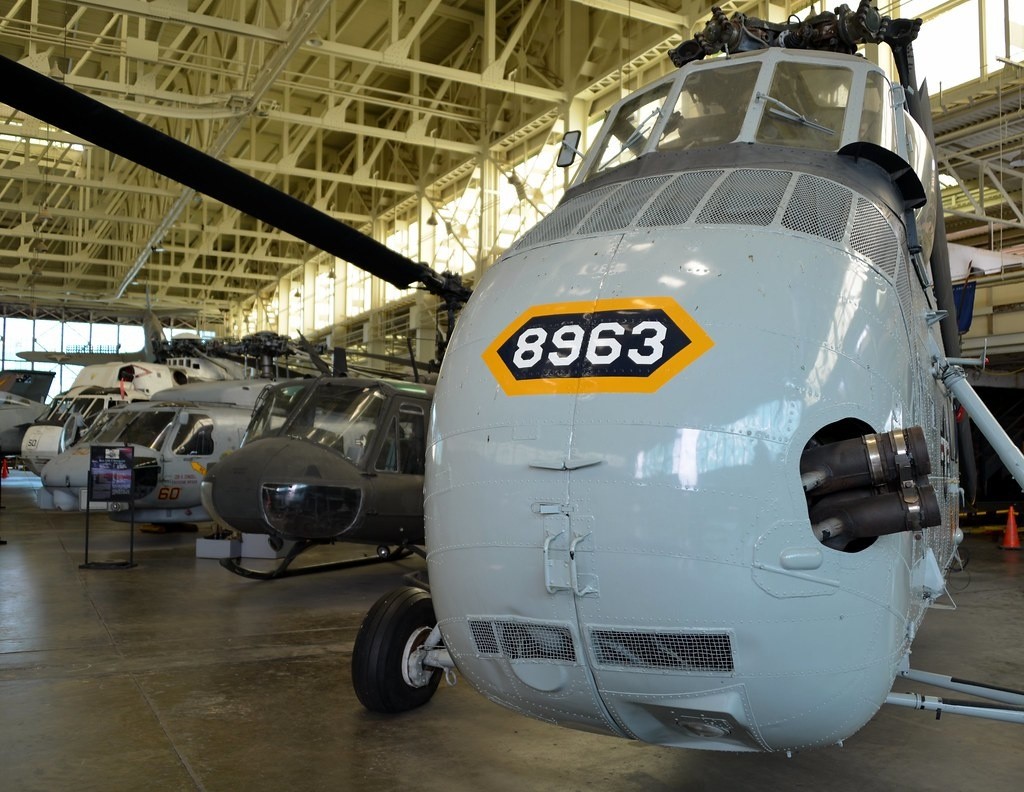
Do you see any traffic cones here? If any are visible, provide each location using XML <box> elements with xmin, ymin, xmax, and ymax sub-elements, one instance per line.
<box><xmin>997</xmin><ymin>505</ymin><xmax>1024</xmax><ymax>552</ymax></box>
<box><xmin>0</xmin><ymin>458</ymin><xmax>10</xmax><ymax>479</ymax></box>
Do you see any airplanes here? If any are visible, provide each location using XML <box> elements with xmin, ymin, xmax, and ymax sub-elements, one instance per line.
<box><xmin>0</xmin><ymin>300</ymin><xmax>442</xmax><ymax>581</ymax></box>
<box><xmin>0</xmin><ymin>0</ymin><xmax>1024</xmax><ymax>760</ymax></box>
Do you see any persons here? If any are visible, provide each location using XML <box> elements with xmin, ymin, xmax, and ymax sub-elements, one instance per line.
<box><xmin>375</xmin><ymin>415</ymin><xmax>418</xmax><ymax>474</ymax></box>
